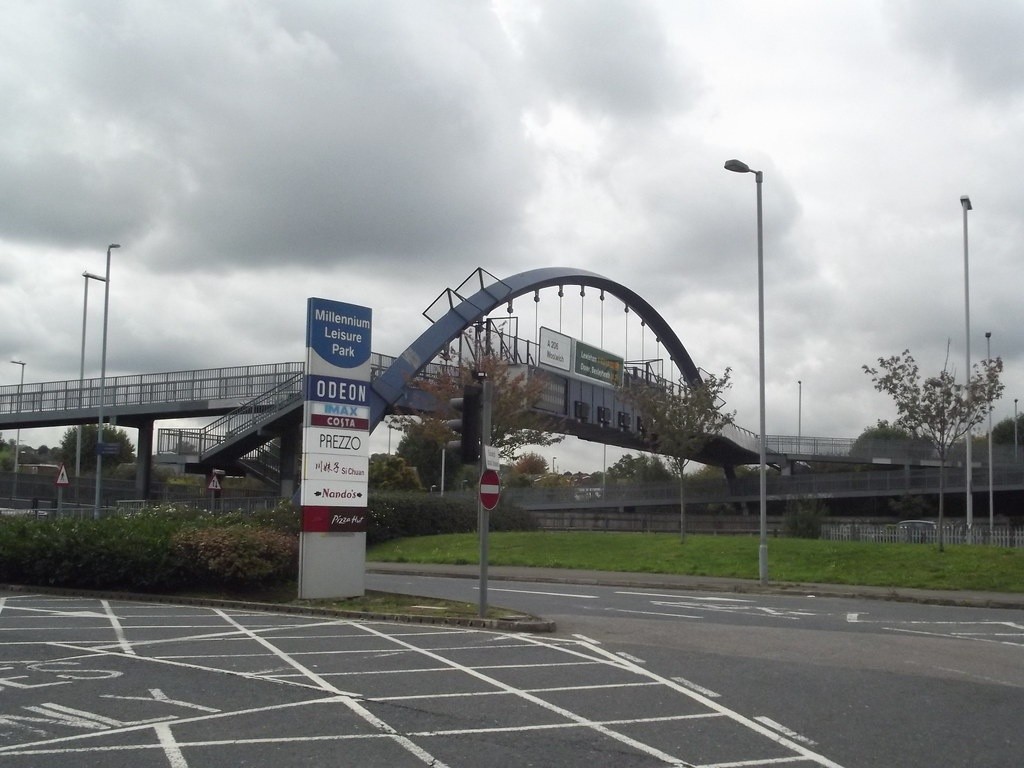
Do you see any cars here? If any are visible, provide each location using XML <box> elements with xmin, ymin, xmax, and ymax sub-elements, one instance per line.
<box><xmin>880</xmin><ymin>518</ymin><xmax>951</xmax><ymax>538</ymax></box>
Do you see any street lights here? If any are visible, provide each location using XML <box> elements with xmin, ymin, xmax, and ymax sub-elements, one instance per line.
<box><xmin>74</xmin><ymin>271</ymin><xmax>110</xmax><ymax>503</ymax></box>
<box><xmin>9</xmin><ymin>359</ymin><xmax>28</xmax><ymax>499</ymax></box>
<box><xmin>797</xmin><ymin>380</ymin><xmax>802</xmax><ymax>454</ymax></box>
<box><xmin>1013</xmin><ymin>398</ymin><xmax>1019</xmax><ymax>462</ymax></box>
<box><xmin>958</xmin><ymin>192</ymin><xmax>976</xmax><ymax>531</ymax></box>
<box><xmin>553</xmin><ymin>456</ymin><xmax>557</xmax><ymax>474</ymax></box>
<box><xmin>985</xmin><ymin>331</ymin><xmax>994</xmax><ymax>544</ymax></box>
<box><xmin>721</xmin><ymin>157</ymin><xmax>771</xmax><ymax>585</ymax></box>
<box><xmin>93</xmin><ymin>243</ymin><xmax>123</xmax><ymax>520</ymax></box>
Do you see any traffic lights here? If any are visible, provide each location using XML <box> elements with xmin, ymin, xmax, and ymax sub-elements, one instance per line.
<box><xmin>446</xmin><ymin>381</ymin><xmax>484</xmax><ymax>464</ymax></box>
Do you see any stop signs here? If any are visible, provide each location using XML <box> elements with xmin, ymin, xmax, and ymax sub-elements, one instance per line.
<box><xmin>478</xmin><ymin>470</ymin><xmax>501</xmax><ymax>511</ymax></box>
